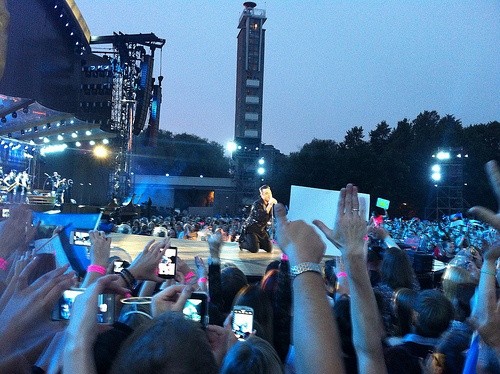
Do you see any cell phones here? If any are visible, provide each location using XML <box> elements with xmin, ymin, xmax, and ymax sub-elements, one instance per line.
<box><xmin>232</xmin><ymin>306</ymin><xmax>254</xmax><ymax>343</ymax></box>
<box><xmin>69</xmin><ymin>231</ymin><xmax>92</xmax><ymax>246</ymax></box>
<box><xmin>50</xmin><ymin>287</ymin><xmax>115</xmax><ymax>326</ymax></box>
<box><xmin>157</xmin><ymin>247</ymin><xmax>177</xmax><ymax>279</ymax></box>
<box><xmin>176</xmin><ymin>292</ymin><xmax>211</xmax><ymax>328</ymax></box>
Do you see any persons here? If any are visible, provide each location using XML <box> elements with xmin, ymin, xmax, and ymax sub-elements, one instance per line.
<box><xmin>238</xmin><ymin>185</ymin><xmax>278</xmax><ymax>253</ymax></box>
<box><xmin>114</xmin><ymin>284</ymin><xmax>220</xmax><ymax>374</ymax></box>
<box><xmin>53</xmin><ymin>175</ymin><xmax>64</xmax><ymax>204</ymax></box>
<box><xmin>4</xmin><ymin>169</ymin><xmax>31</xmax><ymax>204</ymax></box>
<box><xmin>1</xmin><ymin>184</ymin><xmax>500</xmax><ymax>374</ymax></box>
<box><xmin>220</xmin><ymin>336</ymin><xmax>283</xmax><ymax>374</ymax></box>
<box><xmin>98</xmin><ymin>212</ymin><xmax>246</xmax><ymax>242</ymax></box>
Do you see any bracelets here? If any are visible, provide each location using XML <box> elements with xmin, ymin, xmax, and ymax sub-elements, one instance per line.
<box><xmin>120</xmin><ymin>269</ymin><xmax>135</xmax><ymax>287</ymax></box>
<box><xmin>291</xmin><ymin>263</ymin><xmax>323</xmax><ymax>279</ymax></box>
<box><xmin>481</xmin><ymin>272</ymin><xmax>496</xmax><ymax>276</ymax></box>
<box><xmin>88</xmin><ymin>265</ymin><xmax>106</xmax><ymax>275</ymax></box>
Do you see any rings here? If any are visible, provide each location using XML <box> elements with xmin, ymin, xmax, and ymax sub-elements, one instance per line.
<box><xmin>353</xmin><ymin>209</ymin><xmax>359</xmax><ymax>211</ymax></box>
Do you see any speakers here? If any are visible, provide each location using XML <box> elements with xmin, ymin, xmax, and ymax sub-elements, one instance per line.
<box><xmin>403</xmin><ymin>249</ymin><xmax>433</xmax><ymax>274</ymax></box>
<box><xmin>133</xmin><ymin>55</ymin><xmax>161</xmax><ymax>147</ymax></box>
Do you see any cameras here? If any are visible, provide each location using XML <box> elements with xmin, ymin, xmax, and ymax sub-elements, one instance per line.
<box><xmin>113</xmin><ymin>261</ymin><xmax>125</xmax><ymax>273</ymax></box>
<box><xmin>1</xmin><ymin>208</ymin><xmax>11</xmax><ymax>217</ymax></box>
